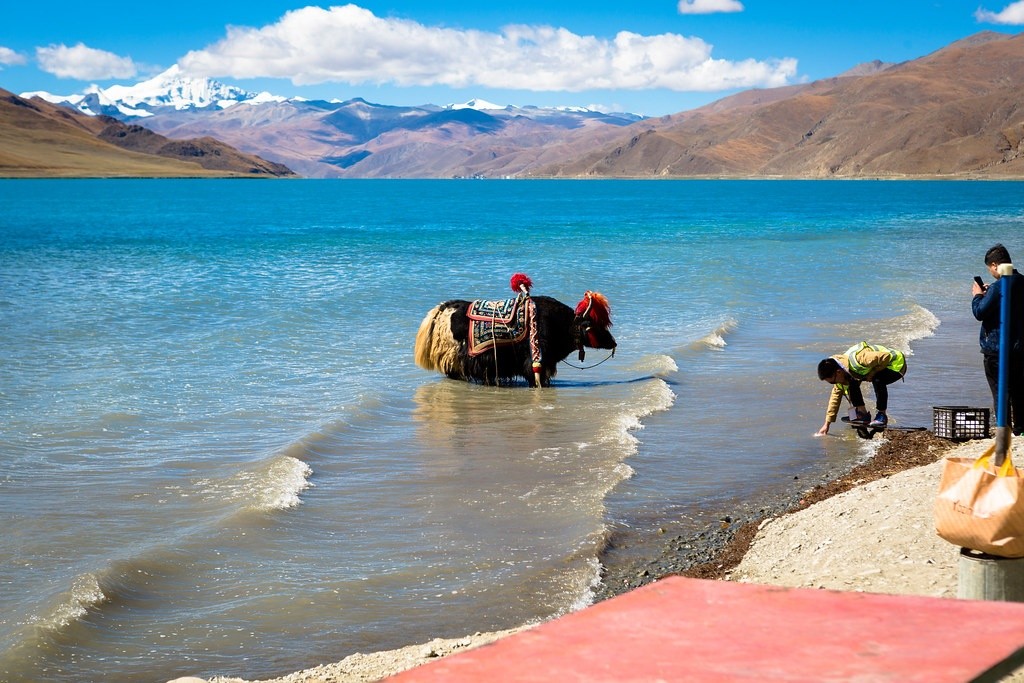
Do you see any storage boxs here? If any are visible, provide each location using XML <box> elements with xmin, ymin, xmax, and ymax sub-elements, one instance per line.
<box><xmin>933</xmin><ymin>405</ymin><xmax>991</xmax><ymax>444</ymax></box>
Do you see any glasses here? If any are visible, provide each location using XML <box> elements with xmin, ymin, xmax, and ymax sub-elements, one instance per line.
<box><xmin>829</xmin><ymin>374</ymin><xmax>837</xmax><ymax>384</ymax></box>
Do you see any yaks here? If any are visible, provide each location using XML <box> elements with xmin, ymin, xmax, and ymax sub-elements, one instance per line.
<box><xmin>409</xmin><ymin>291</ymin><xmax>618</xmax><ymax>392</ymax></box>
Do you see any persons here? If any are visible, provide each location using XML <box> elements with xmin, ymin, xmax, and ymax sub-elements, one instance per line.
<box><xmin>818</xmin><ymin>341</ymin><xmax>907</xmax><ymax>436</ymax></box>
<box><xmin>972</xmin><ymin>244</ymin><xmax>1024</xmax><ymax>438</ymax></box>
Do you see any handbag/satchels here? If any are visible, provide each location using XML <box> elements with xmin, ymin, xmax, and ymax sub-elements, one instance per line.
<box><xmin>934</xmin><ymin>443</ymin><xmax>1024</xmax><ymax>558</ymax></box>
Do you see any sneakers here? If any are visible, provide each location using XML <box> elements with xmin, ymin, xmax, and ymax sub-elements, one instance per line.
<box><xmin>841</xmin><ymin>410</ymin><xmax>888</xmax><ymax>427</ymax></box>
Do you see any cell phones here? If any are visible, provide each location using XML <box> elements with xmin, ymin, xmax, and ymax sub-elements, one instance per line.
<box><xmin>974</xmin><ymin>276</ymin><xmax>986</xmax><ymax>292</ymax></box>
